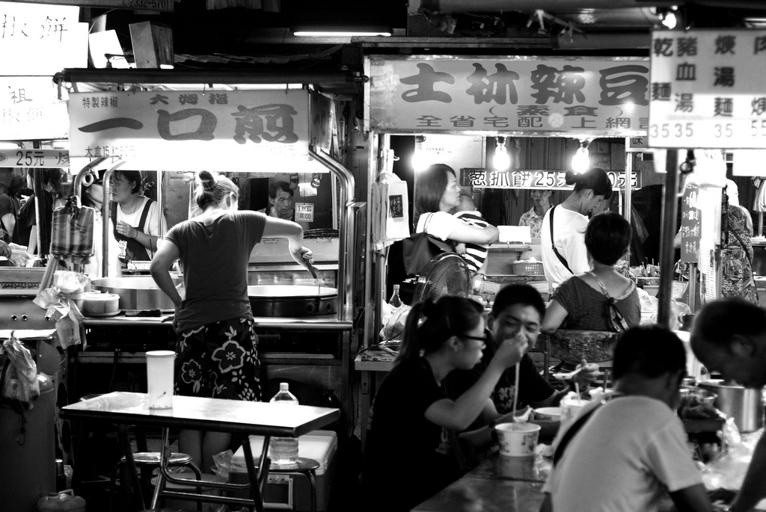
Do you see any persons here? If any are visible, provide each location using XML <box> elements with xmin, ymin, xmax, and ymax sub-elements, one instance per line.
<box><xmin>416</xmin><ymin>164</ymin><xmax>758</xmax><ymax>333</ymax></box>
<box><xmin>362</xmin><ymin>296</ymin><xmax>529</xmax><ymax>512</ymax></box>
<box><xmin>446</xmin><ymin>285</ymin><xmax>600</xmax><ymax>471</ymax></box>
<box><xmin>150</xmin><ymin>170</ymin><xmax>314</xmax><ymax>474</ymax></box>
<box><xmin>690</xmin><ymin>298</ymin><xmax>766</xmax><ymax>512</ymax></box>
<box><xmin>539</xmin><ymin>326</ymin><xmax>716</xmax><ymax>512</ymax></box>
<box><xmin>0</xmin><ymin>170</ymin><xmax>309</xmax><ymax>274</ymax></box>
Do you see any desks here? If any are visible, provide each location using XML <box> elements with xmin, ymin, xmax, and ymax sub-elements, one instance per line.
<box><xmin>354</xmin><ymin>345</ymin><xmax>401</xmax><ymax>451</ymax></box>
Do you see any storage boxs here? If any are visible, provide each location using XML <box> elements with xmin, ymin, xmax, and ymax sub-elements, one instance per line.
<box><xmin>227</xmin><ymin>430</ymin><xmax>337</xmax><ymax>511</ymax></box>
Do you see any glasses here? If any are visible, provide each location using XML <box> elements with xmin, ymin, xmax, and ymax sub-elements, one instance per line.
<box><xmin>464</xmin><ymin>329</ymin><xmax>491</xmax><ymax>345</ymax></box>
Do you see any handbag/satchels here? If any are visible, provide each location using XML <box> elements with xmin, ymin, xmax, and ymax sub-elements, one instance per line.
<box><xmin>387</xmin><ymin>232</ymin><xmax>456</xmax><ymax>293</ymax></box>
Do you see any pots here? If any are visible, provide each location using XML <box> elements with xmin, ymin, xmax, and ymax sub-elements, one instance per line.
<box><xmin>92</xmin><ymin>275</ymin><xmax>178</xmax><ymax>313</ymax></box>
<box><xmin>245</xmin><ymin>283</ymin><xmax>337</xmax><ymax>319</ymax></box>
<box><xmin>701</xmin><ymin>380</ymin><xmax>761</xmax><ymax>433</ymax></box>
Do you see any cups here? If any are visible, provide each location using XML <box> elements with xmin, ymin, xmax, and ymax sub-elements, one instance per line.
<box><xmin>146</xmin><ymin>350</ymin><xmax>175</xmax><ymax>409</ymax></box>
<box><xmin>560</xmin><ymin>398</ymin><xmax>588</xmax><ymax>421</ymax></box>
<box><xmin>586</xmin><ymin>386</ymin><xmax>613</xmax><ymax>399</ymax></box>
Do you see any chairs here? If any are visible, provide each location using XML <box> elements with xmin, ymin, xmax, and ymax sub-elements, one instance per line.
<box><xmin>536</xmin><ymin>332</ymin><xmax>620</xmax><ymax>385</ymax></box>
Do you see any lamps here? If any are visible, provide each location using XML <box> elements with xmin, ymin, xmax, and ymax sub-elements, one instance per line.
<box><xmin>410</xmin><ymin>135</ymin><xmax>432</xmax><ymax>171</ymax></box>
<box><xmin>490</xmin><ymin>138</ymin><xmax>512</xmax><ymax>172</ymax></box>
<box><xmin>570</xmin><ymin>141</ymin><xmax>592</xmax><ymax>173</ymax></box>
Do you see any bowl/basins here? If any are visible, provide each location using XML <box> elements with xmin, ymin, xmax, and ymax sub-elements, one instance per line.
<box><xmin>533</xmin><ymin>406</ymin><xmax>561</xmax><ymax>423</ymax></box>
<box><xmin>494</xmin><ymin>421</ymin><xmax>542</xmax><ymax>457</ymax></box>
<box><xmin>75</xmin><ymin>295</ymin><xmax>119</xmax><ymax>317</ymax></box>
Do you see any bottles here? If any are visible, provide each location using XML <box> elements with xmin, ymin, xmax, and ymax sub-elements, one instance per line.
<box><xmin>269</xmin><ymin>382</ymin><xmax>300</xmax><ymax>464</ymax></box>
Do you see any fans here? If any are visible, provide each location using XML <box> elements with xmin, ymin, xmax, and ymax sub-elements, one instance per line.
<box><xmin>414</xmin><ymin>253</ymin><xmax>473</xmax><ymax>308</ymax></box>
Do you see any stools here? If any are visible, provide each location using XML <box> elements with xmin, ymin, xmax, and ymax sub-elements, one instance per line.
<box><xmin>109</xmin><ymin>453</ymin><xmax>203</xmax><ymax>512</ymax></box>
<box><xmin>248</xmin><ymin>457</ymin><xmax>319</xmax><ymax>512</ymax></box>
